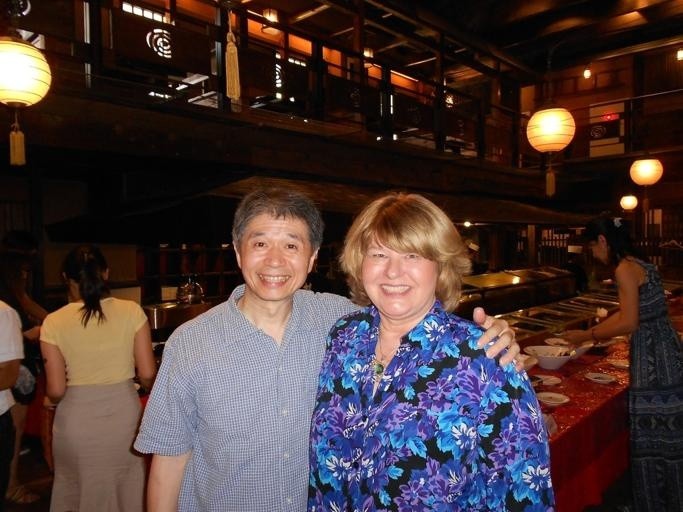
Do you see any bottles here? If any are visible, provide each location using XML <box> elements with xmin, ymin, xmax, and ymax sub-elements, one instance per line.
<box><xmin>176</xmin><ymin>272</ymin><xmax>202</xmax><ymax>304</ymax></box>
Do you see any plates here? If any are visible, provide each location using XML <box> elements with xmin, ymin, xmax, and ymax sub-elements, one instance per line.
<box><xmin>609</xmin><ymin>358</ymin><xmax>631</xmax><ymax>369</ymax></box>
<box><xmin>587</xmin><ymin>373</ymin><xmax>614</xmax><ymax>384</ymax></box>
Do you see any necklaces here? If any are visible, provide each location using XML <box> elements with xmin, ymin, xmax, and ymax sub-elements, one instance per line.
<box><xmin>372</xmin><ymin>327</ymin><xmax>398</xmax><ymax>379</ymax></box>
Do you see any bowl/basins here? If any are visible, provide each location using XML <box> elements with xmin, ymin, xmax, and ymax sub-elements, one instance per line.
<box><xmin>519</xmin><ymin>346</ymin><xmax>576</xmax><ymax>406</ymax></box>
<box><xmin>614</xmin><ymin>335</ymin><xmax>627</xmax><ymax>342</ymax></box>
<box><xmin>591</xmin><ymin>339</ymin><xmax>618</xmax><ymax>351</ymax></box>
<box><xmin>543</xmin><ymin>337</ymin><xmax>593</xmax><ymax>361</ymax></box>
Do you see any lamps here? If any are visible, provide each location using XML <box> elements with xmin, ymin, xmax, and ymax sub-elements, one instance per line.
<box><xmin>363</xmin><ymin>32</ymin><xmax>374</xmax><ymax>68</ymax></box>
<box><xmin>0</xmin><ymin>0</ymin><xmax>52</xmax><ymax>166</ymax></box>
<box><xmin>629</xmin><ymin>115</ymin><xmax>664</xmax><ymax>212</ymax></box>
<box><xmin>262</xmin><ymin>0</ymin><xmax>280</xmax><ymax>34</ymax></box>
<box><xmin>527</xmin><ymin>74</ymin><xmax>576</xmax><ymax>197</ymax></box>
<box><xmin>619</xmin><ymin>193</ymin><xmax>639</xmax><ymax>213</ymax></box>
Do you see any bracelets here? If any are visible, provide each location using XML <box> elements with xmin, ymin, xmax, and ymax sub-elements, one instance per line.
<box><xmin>592</xmin><ymin>327</ymin><xmax>598</xmax><ymax>341</ymax></box>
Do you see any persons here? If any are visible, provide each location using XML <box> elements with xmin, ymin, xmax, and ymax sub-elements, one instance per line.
<box><xmin>132</xmin><ymin>184</ymin><xmax>526</xmax><ymax>512</ymax></box>
<box><xmin>39</xmin><ymin>244</ymin><xmax>157</xmax><ymax>512</ymax></box>
<box><xmin>559</xmin><ymin>217</ymin><xmax>683</xmax><ymax>512</ymax></box>
<box><xmin>307</xmin><ymin>192</ymin><xmax>557</xmax><ymax>512</ymax></box>
<box><xmin>0</xmin><ymin>300</ymin><xmax>26</xmax><ymax>512</ymax></box>
<box><xmin>0</xmin><ymin>229</ymin><xmax>50</xmax><ymax>508</ymax></box>
